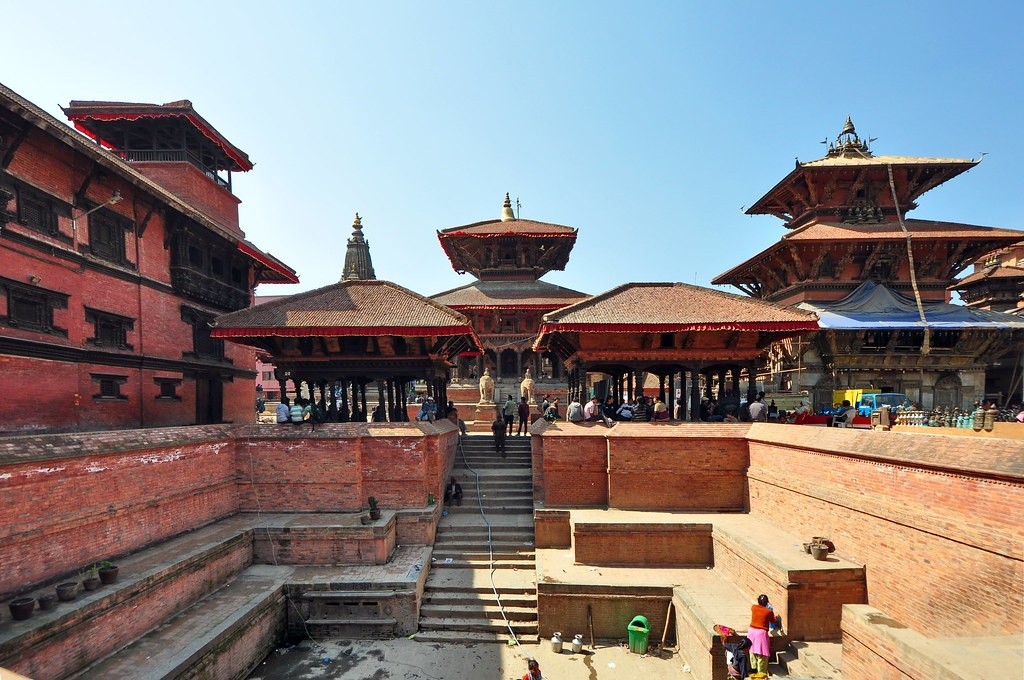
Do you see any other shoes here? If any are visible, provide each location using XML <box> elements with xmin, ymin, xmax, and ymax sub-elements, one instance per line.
<box><xmin>611</xmin><ymin>422</ymin><xmax>616</xmax><ymax>428</ymax></box>
<box><xmin>415</xmin><ymin>416</ymin><xmax>420</xmax><ymax>422</ymax></box>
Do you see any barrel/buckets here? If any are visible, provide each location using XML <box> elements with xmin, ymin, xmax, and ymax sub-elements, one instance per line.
<box><xmin>572</xmin><ymin>634</ymin><xmax>583</xmax><ymax>653</ymax></box>
<box><xmin>803</xmin><ymin>537</ymin><xmax>830</xmax><ymax>560</ymax></box>
<box><xmin>551</xmin><ymin>632</ymin><xmax>563</xmax><ymax>653</ymax></box>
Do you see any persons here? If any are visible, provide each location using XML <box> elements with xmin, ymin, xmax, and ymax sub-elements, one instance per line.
<box><xmin>542</xmin><ymin>394</ymin><xmax>990</xmax><ymax>427</ymax></box>
<box><xmin>523</xmin><ymin>660</ymin><xmax>542</xmax><ymax>680</ymax></box>
<box><xmin>276</xmin><ymin>397</ymin><xmax>467</xmax><ymax>438</ymax></box>
<box><xmin>446</xmin><ymin>477</ymin><xmax>463</xmax><ymax>507</ymax></box>
<box><xmin>502</xmin><ymin>395</ymin><xmax>515</xmax><ymax>437</ymax></box>
<box><xmin>491</xmin><ymin>414</ymin><xmax>506</xmax><ymax>457</ymax></box>
<box><xmin>515</xmin><ymin>397</ymin><xmax>530</xmax><ymax>437</ymax></box>
<box><xmin>747</xmin><ymin>594</ymin><xmax>777</xmax><ymax>674</ymax></box>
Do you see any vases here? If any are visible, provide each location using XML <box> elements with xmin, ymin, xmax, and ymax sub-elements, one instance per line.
<box><xmin>8</xmin><ymin>597</ymin><xmax>35</xmax><ymax>620</ymax></box>
<box><xmin>54</xmin><ymin>581</ymin><xmax>80</xmax><ymax>601</ymax></box>
<box><xmin>803</xmin><ymin>534</ymin><xmax>831</xmax><ymax>561</ymax></box>
<box><xmin>37</xmin><ymin>594</ymin><xmax>57</xmax><ymax>611</ymax></box>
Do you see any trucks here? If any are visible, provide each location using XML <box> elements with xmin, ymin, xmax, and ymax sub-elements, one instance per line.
<box><xmin>833</xmin><ymin>389</ymin><xmax>911</xmax><ymax>418</ymax></box>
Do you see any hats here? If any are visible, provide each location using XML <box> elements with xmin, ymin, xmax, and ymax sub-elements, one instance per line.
<box><xmin>702</xmin><ymin>397</ymin><xmax>709</xmax><ymax>401</ymax></box>
<box><xmin>802</xmin><ymin>400</ymin><xmax>808</xmax><ymax>406</ymax></box>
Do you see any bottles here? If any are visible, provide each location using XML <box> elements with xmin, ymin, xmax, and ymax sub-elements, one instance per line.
<box><xmin>442</xmin><ymin>511</ymin><xmax>448</xmax><ymax>516</ymax></box>
<box><xmin>429</xmin><ymin>491</ymin><xmax>434</xmax><ymax>505</ymax></box>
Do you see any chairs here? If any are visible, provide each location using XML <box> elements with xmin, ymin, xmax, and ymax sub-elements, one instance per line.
<box><xmin>831</xmin><ymin>409</ymin><xmax>859</xmax><ymax>430</ymax></box>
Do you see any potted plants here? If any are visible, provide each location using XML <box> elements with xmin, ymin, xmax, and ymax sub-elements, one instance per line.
<box><xmin>359</xmin><ymin>511</ymin><xmax>371</xmax><ymax>525</ymax></box>
<box><xmin>368</xmin><ymin>494</ymin><xmax>382</xmax><ymax>520</ymax></box>
<box><xmin>82</xmin><ymin>566</ymin><xmax>99</xmax><ymax>591</ymax></box>
<box><xmin>98</xmin><ymin>559</ymin><xmax>120</xmax><ymax>584</ymax></box>
